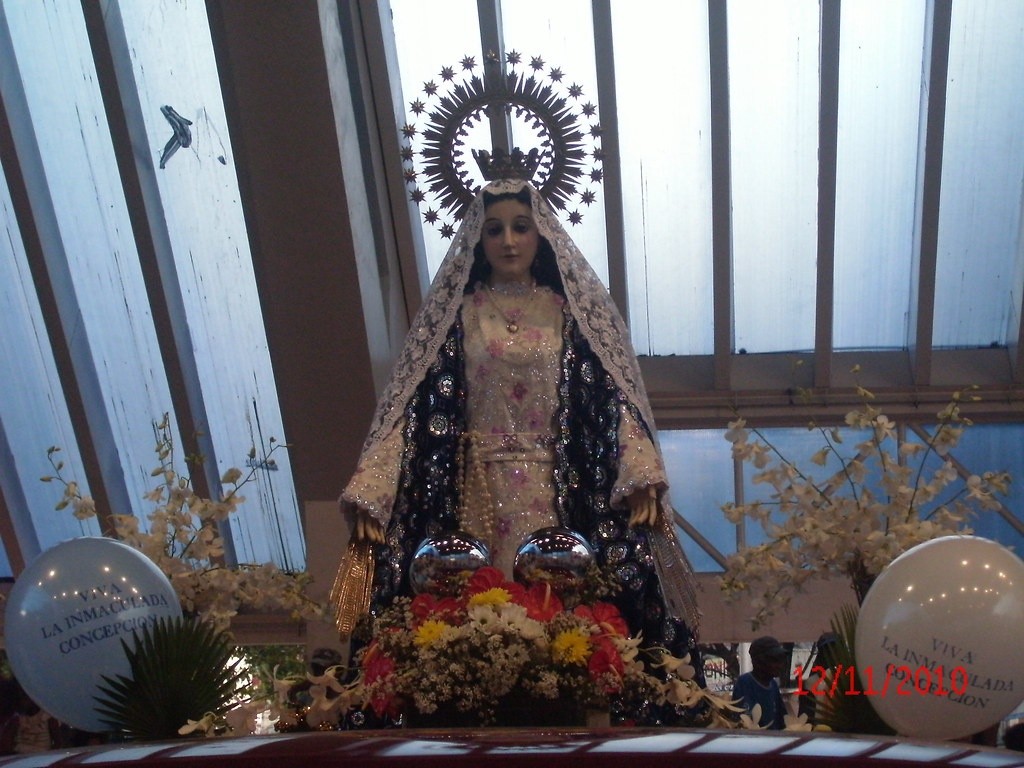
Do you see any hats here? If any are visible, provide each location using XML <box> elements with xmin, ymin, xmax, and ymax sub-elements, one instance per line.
<box><xmin>817</xmin><ymin>632</ymin><xmax>845</xmax><ymax>652</ymax></box>
<box><xmin>750</xmin><ymin>636</ymin><xmax>787</xmax><ymax>662</ymax></box>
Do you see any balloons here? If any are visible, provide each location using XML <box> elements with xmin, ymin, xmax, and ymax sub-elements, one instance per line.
<box><xmin>853</xmin><ymin>535</ymin><xmax>1024</xmax><ymax>740</ymax></box>
<box><xmin>4</xmin><ymin>537</ymin><xmax>183</xmax><ymax>735</ymax></box>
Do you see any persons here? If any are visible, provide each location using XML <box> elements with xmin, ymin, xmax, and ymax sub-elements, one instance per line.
<box><xmin>816</xmin><ymin>632</ymin><xmax>847</xmax><ymax>670</ymax></box>
<box><xmin>730</xmin><ymin>637</ymin><xmax>789</xmax><ymax>729</ymax></box>
<box><xmin>328</xmin><ymin>149</ymin><xmax>707</xmax><ymax>730</ymax></box>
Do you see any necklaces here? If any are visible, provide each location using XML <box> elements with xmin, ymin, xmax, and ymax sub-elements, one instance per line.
<box><xmin>489</xmin><ymin>277</ymin><xmax>537</xmax><ymax>332</ymax></box>
<box><xmin>459</xmin><ymin>430</ymin><xmax>493</xmax><ymax>542</ymax></box>
